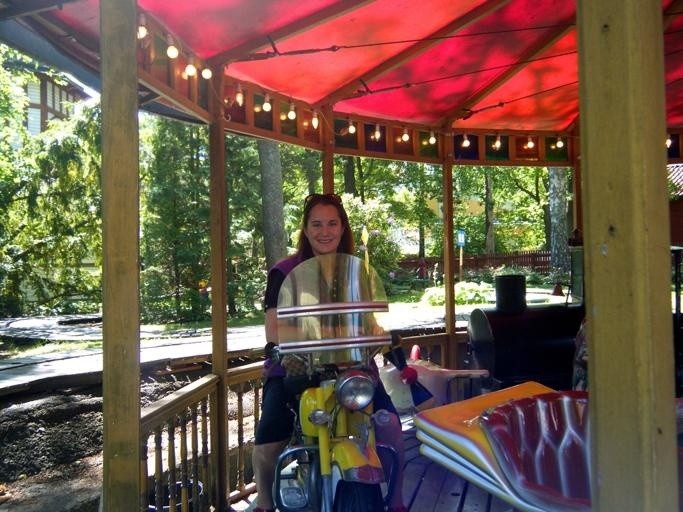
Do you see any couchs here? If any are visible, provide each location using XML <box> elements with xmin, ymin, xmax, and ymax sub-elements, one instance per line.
<box><xmin>468</xmin><ymin>306</ymin><xmax>588</xmax><ymax>391</ymax></box>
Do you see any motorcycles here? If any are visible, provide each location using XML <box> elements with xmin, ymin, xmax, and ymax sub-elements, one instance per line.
<box><xmin>260</xmin><ymin>253</ymin><xmax>406</xmax><ymax>512</ymax></box>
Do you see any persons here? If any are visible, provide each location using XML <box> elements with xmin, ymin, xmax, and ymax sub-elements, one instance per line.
<box><xmin>568</xmin><ymin>229</ymin><xmax>583</xmax><ymax>247</ymax></box>
<box><xmin>251</xmin><ymin>193</ymin><xmax>412</xmax><ymax>511</ymax></box>
<box><xmin>417</xmin><ymin>256</ymin><xmax>426</xmax><ymax>277</ymax></box>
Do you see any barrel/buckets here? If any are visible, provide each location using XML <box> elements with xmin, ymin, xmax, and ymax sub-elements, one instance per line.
<box><xmin>494</xmin><ymin>274</ymin><xmax>527</xmax><ymax>314</ymax></box>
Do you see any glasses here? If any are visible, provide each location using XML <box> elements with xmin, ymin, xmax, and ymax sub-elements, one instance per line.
<box><xmin>304</xmin><ymin>193</ymin><xmax>342</xmax><ymax>209</ymax></box>
